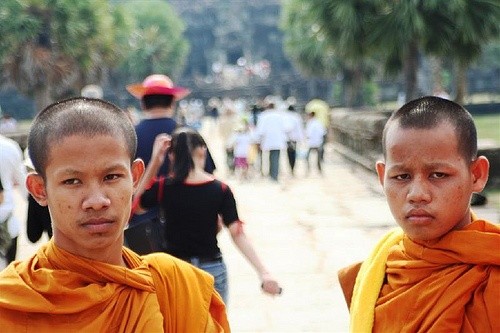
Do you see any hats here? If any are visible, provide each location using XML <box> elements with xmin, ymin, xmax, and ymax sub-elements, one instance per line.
<box><xmin>126</xmin><ymin>74</ymin><xmax>191</xmax><ymax>101</ymax></box>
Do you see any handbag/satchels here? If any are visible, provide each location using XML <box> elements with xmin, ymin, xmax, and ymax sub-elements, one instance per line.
<box><xmin>123</xmin><ymin>176</ymin><xmax>168</xmax><ymax>254</ymax></box>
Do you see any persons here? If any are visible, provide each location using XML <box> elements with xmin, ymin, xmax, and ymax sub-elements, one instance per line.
<box><xmin>0</xmin><ymin>96</ymin><xmax>231</xmax><ymax>333</ymax></box>
<box><xmin>337</xmin><ymin>96</ymin><xmax>500</xmax><ymax>333</ymax></box>
<box><xmin>0</xmin><ymin>74</ymin><xmax>329</xmax><ymax>304</ymax></box>
